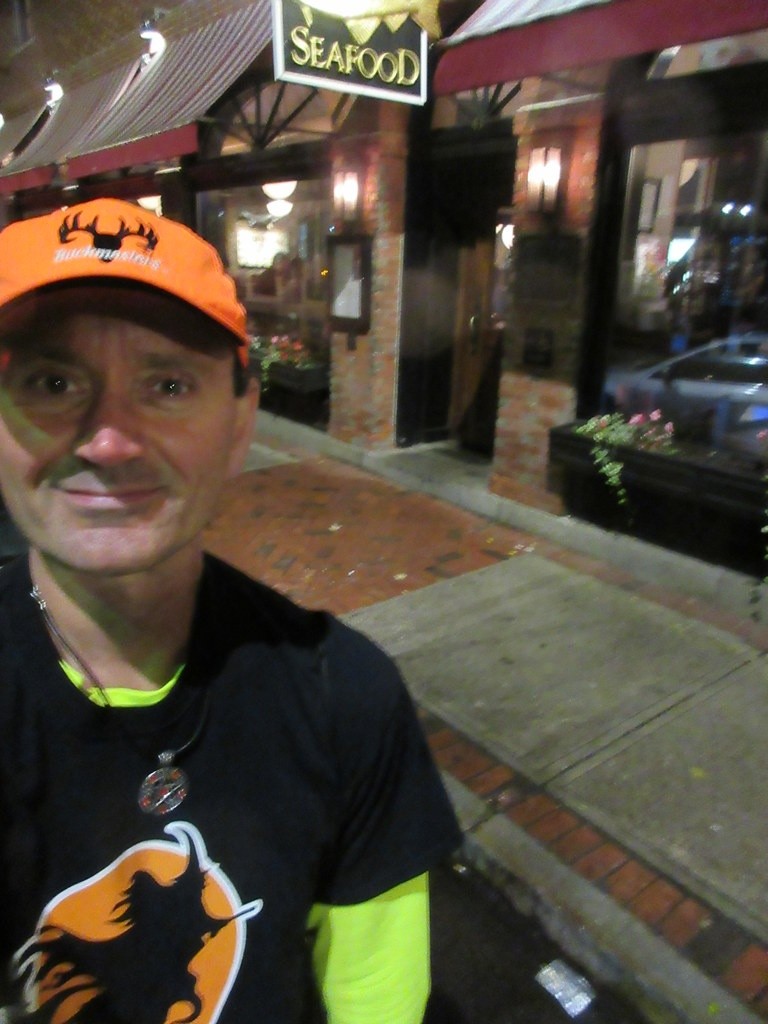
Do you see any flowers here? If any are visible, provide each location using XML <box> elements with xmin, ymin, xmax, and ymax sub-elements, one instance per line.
<box><xmin>577</xmin><ymin>407</ymin><xmax>674</xmax><ymax>505</ymax></box>
<box><xmin>746</xmin><ymin>429</ymin><xmax>768</xmax><ymax>623</ymax></box>
<box><xmin>245</xmin><ymin>333</ymin><xmax>315</xmax><ymax>391</ymax></box>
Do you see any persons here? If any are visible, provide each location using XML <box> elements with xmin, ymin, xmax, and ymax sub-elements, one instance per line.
<box><xmin>0</xmin><ymin>197</ymin><xmax>469</xmax><ymax>1024</ymax></box>
<box><xmin>254</xmin><ymin>252</ymin><xmax>312</xmax><ymax>298</ymax></box>
<box><xmin>663</xmin><ymin>258</ymin><xmax>687</xmax><ymax>320</ymax></box>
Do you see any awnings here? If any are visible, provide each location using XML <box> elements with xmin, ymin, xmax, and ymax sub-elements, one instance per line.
<box><xmin>0</xmin><ymin>0</ymin><xmax>273</xmax><ymax>195</ymax></box>
<box><xmin>0</xmin><ymin>104</ymin><xmax>47</xmax><ymax>160</ymax></box>
<box><xmin>432</xmin><ymin>0</ymin><xmax>768</xmax><ymax>97</ymax></box>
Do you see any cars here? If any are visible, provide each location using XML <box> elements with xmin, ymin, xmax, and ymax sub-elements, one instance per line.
<box><xmin>590</xmin><ymin>329</ymin><xmax>768</xmax><ymax>462</ymax></box>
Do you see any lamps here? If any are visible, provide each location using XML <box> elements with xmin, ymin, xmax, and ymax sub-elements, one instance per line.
<box><xmin>527</xmin><ymin>129</ymin><xmax>567</xmax><ymax>215</ymax></box>
<box><xmin>140</xmin><ymin>7</ymin><xmax>166</xmax><ymax>41</ymax></box>
<box><xmin>44</xmin><ymin>76</ymin><xmax>57</xmax><ymax>92</ymax></box>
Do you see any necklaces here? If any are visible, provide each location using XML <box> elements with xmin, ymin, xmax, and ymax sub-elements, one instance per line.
<box><xmin>30</xmin><ymin>586</ymin><xmax>212</xmax><ymax>815</ymax></box>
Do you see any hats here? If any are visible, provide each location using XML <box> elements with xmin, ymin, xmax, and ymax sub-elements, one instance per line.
<box><xmin>0</xmin><ymin>197</ymin><xmax>249</xmax><ymax>371</ymax></box>
